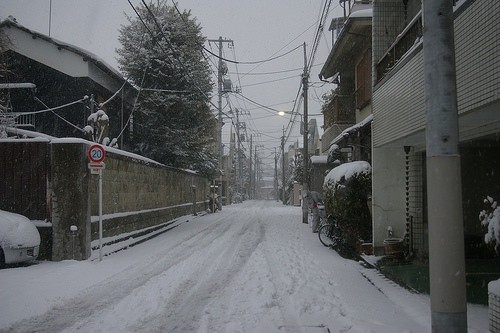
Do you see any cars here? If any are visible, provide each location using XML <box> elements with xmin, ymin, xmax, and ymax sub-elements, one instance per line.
<box><xmin>0</xmin><ymin>210</ymin><xmax>40</xmax><ymax>271</ymax></box>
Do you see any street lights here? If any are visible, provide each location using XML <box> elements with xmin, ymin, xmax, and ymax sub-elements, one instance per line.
<box><xmin>279</xmin><ymin>110</ymin><xmax>309</xmax><ymax>205</ymax></box>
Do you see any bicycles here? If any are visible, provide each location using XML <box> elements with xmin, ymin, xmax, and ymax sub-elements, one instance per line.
<box><xmin>318</xmin><ymin>211</ymin><xmax>342</xmax><ymax>249</ymax></box>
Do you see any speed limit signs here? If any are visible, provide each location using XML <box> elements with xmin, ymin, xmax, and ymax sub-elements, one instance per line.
<box><xmin>88</xmin><ymin>144</ymin><xmax>105</xmax><ymax>162</ymax></box>
